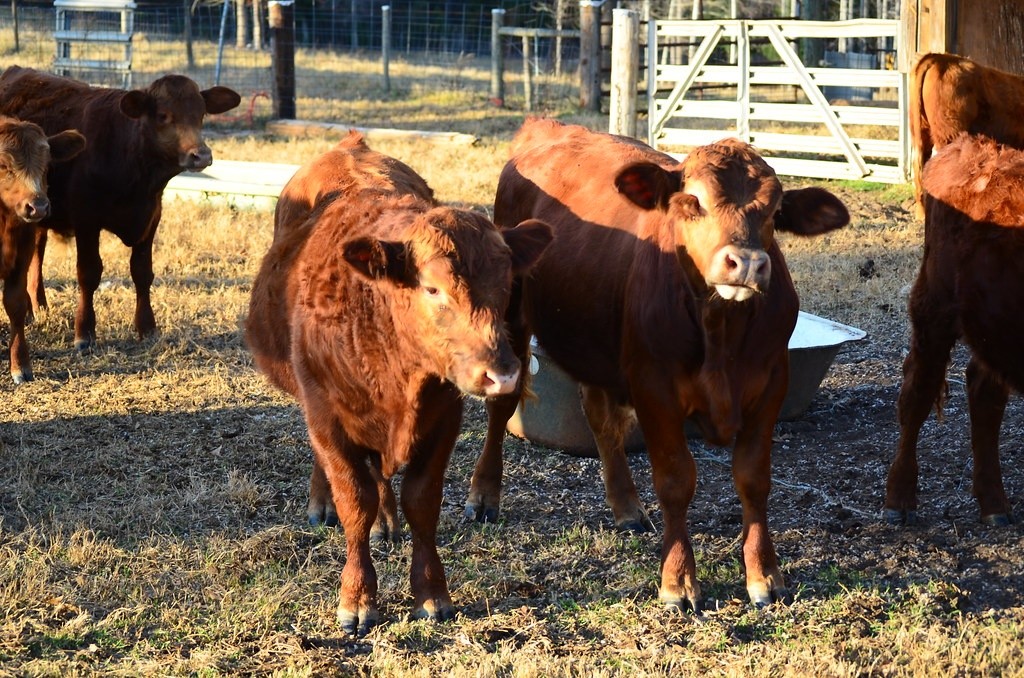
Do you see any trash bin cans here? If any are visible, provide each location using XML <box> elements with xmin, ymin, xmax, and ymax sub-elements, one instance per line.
<box><xmin>821</xmin><ymin>51</ymin><xmax>878</xmax><ymax>101</ymax></box>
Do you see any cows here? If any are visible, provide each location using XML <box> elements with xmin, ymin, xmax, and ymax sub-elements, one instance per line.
<box><xmin>875</xmin><ymin>50</ymin><xmax>1024</xmax><ymax>528</ymax></box>
<box><xmin>459</xmin><ymin>113</ymin><xmax>853</xmax><ymax>613</ymax></box>
<box><xmin>0</xmin><ymin>64</ymin><xmax>241</xmax><ymax>384</ymax></box>
<box><xmin>242</xmin><ymin>129</ymin><xmax>556</xmax><ymax>636</ymax></box>
